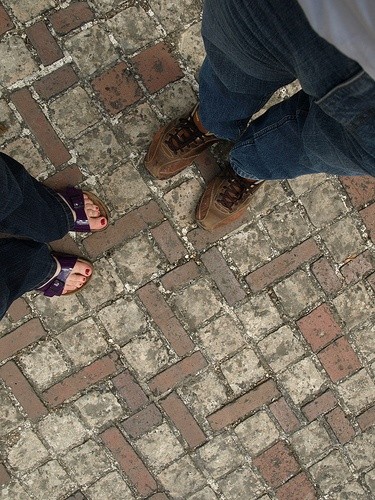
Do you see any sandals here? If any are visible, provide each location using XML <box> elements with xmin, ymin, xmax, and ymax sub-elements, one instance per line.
<box><xmin>32</xmin><ymin>248</ymin><xmax>93</xmax><ymax>301</ymax></box>
<box><xmin>52</xmin><ymin>184</ymin><xmax>110</xmax><ymax>234</ymax></box>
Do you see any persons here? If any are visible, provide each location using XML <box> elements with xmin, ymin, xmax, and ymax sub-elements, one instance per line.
<box><xmin>0</xmin><ymin>151</ymin><xmax>111</xmax><ymax>323</ymax></box>
<box><xmin>142</xmin><ymin>0</ymin><xmax>375</xmax><ymax>231</ymax></box>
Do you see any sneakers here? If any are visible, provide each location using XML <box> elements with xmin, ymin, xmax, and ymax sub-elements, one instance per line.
<box><xmin>194</xmin><ymin>162</ymin><xmax>263</xmax><ymax>236</ymax></box>
<box><xmin>144</xmin><ymin>105</ymin><xmax>232</xmax><ymax>183</ymax></box>
